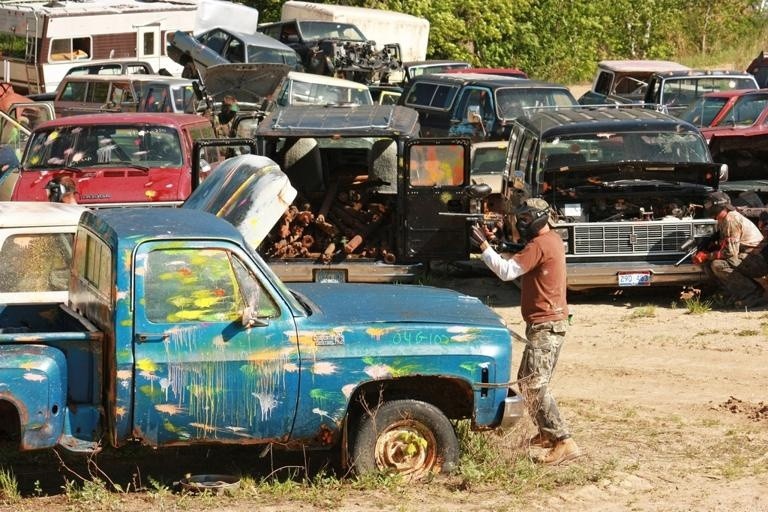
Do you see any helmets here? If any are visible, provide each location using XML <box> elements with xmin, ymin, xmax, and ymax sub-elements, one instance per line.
<box><xmin>706</xmin><ymin>192</ymin><xmax>735</xmax><ymax>215</ymax></box>
<box><xmin>516</xmin><ymin>198</ymin><xmax>552</xmax><ymax>241</ymax></box>
<box><xmin>221</xmin><ymin>97</ymin><xmax>239</xmax><ymax>115</ymax></box>
<box><xmin>43</xmin><ymin>175</ymin><xmax>77</xmax><ymax>202</ymax></box>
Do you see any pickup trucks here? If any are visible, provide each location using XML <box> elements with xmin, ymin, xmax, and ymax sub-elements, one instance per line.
<box><xmin>0</xmin><ymin>202</ymin><xmax>528</xmax><ymax>487</ymax></box>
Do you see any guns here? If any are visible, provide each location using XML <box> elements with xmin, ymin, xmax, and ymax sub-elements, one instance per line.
<box><xmin>437</xmin><ymin>213</ymin><xmax>526</xmax><ymax>252</ymax></box>
<box><xmin>675</xmin><ymin>230</ymin><xmax>721</xmax><ymax>267</ymax></box>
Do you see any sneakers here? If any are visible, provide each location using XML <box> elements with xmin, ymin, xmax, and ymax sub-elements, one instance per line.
<box><xmin>734</xmin><ymin>284</ymin><xmax>765</xmax><ymax>308</ymax></box>
<box><xmin>533</xmin><ymin>436</ymin><xmax>582</xmax><ymax>465</ymax></box>
<box><xmin>521</xmin><ymin>434</ymin><xmax>552</xmax><ymax>448</ymax></box>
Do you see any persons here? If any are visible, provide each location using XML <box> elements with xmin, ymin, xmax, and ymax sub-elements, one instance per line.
<box><xmin>691</xmin><ymin>192</ymin><xmax>768</xmax><ymax>309</ymax></box>
<box><xmin>215</xmin><ymin>95</ymin><xmax>245</xmax><ymax>156</ymax></box>
<box><xmin>46</xmin><ymin>177</ymin><xmax>77</xmax><ymax>205</ymax></box>
<box><xmin>468</xmin><ymin>197</ymin><xmax>580</xmax><ymax>466</ymax></box>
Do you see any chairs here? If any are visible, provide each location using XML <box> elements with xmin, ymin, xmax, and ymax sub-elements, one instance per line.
<box><xmin>548</xmin><ymin>152</ymin><xmax>586</xmax><ymax>187</ymax></box>
<box><xmin>207</xmin><ymin>36</ymin><xmax>226</xmax><ymax>53</ymax></box>
<box><xmin>51</xmin><ymin>137</ymin><xmax>92</xmax><ymax>163</ymax></box>
